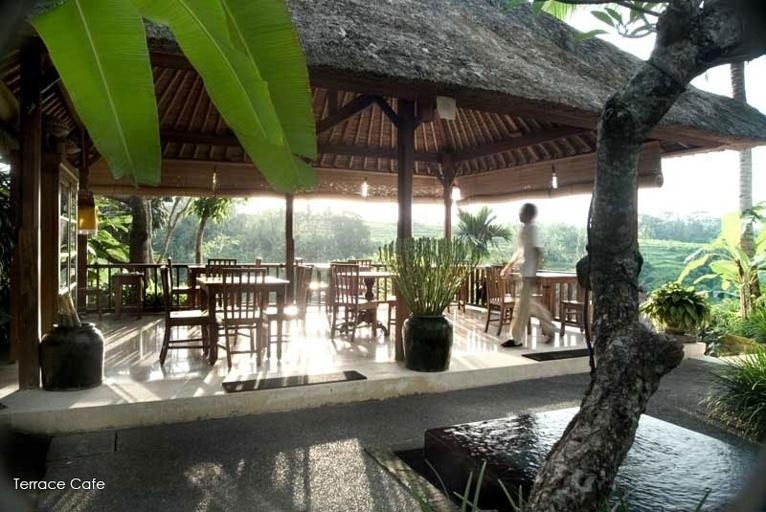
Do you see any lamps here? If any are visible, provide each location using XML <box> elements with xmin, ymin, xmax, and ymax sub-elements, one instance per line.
<box><xmin>551</xmin><ymin>152</ymin><xmax>557</xmax><ymax>188</ymax></box>
<box><xmin>361</xmin><ymin>176</ymin><xmax>368</xmax><ymax>197</ymax></box>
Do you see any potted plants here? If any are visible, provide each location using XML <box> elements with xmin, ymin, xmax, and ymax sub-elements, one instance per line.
<box><xmin>637</xmin><ymin>282</ymin><xmax>712</xmax><ymax>335</ymax></box>
<box><xmin>383</xmin><ymin>232</ymin><xmax>485</xmax><ymax>374</ymax></box>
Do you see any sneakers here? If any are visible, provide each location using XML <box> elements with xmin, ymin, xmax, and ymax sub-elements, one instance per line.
<box><xmin>501</xmin><ymin>339</ymin><xmax>523</xmax><ymax>348</ymax></box>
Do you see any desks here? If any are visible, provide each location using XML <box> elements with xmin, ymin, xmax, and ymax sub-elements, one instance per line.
<box><xmin>112</xmin><ymin>271</ymin><xmax>146</xmax><ymax>322</ymax></box>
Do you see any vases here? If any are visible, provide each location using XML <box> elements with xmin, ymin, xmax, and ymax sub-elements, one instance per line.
<box><xmin>35</xmin><ymin>320</ymin><xmax>108</xmax><ymax>393</ymax></box>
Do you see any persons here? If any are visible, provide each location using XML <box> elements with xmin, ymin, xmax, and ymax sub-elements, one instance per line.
<box><xmin>498</xmin><ymin>202</ymin><xmax>558</xmax><ymax>347</ymax></box>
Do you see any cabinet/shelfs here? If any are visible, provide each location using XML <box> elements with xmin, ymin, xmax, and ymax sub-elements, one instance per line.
<box><xmin>41</xmin><ymin>153</ymin><xmax>80</xmax><ymax>334</ymax></box>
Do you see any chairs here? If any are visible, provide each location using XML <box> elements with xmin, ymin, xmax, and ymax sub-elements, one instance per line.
<box><xmin>160</xmin><ymin>256</ymin><xmax>316</xmax><ymax>366</ymax></box>
<box><xmin>486</xmin><ymin>263</ymin><xmax>594</xmax><ymax>346</ymax></box>
<box><xmin>78</xmin><ymin>262</ymin><xmax>103</xmax><ymax>320</ymax></box>
<box><xmin>327</xmin><ymin>260</ymin><xmax>396</xmax><ymax>344</ymax></box>
<box><xmin>447</xmin><ymin>274</ymin><xmax>467</xmax><ymax>313</ymax></box>
